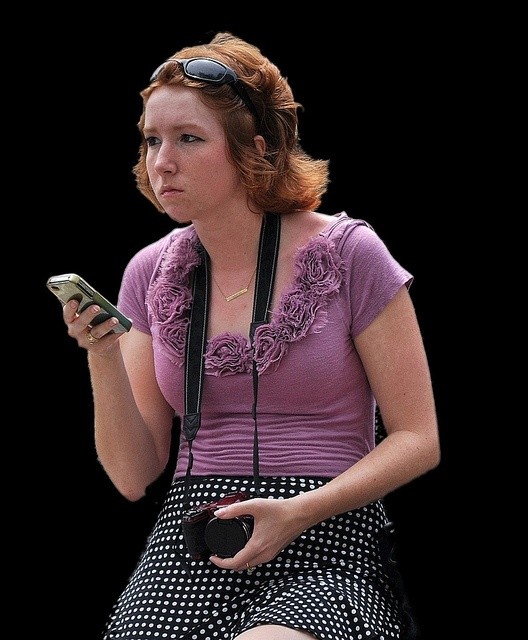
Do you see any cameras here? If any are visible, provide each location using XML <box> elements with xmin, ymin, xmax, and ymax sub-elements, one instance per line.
<box><xmin>182</xmin><ymin>490</ymin><xmax>253</xmax><ymax>561</ymax></box>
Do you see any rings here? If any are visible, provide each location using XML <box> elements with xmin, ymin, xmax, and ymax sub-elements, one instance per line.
<box><xmin>86</xmin><ymin>328</ymin><xmax>99</xmax><ymax>345</ymax></box>
<box><xmin>245</xmin><ymin>562</ymin><xmax>255</xmax><ymax>572</ymax></box>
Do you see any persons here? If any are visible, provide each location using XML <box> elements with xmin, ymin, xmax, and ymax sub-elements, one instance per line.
<box><xmin>63</xmin><ymin>32</ymin><xmax>440</xmax><ymax>640</ymax></box>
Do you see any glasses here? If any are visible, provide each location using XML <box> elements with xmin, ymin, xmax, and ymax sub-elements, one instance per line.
<box><xmin>147</xmin><ymin>56</ymin><xmax>265</xmax><ymax>133</ymax></box>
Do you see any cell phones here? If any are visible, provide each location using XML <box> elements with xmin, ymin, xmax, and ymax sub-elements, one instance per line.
<box><xmin>46</xmin><ymin>273</ymin><xmax>132</xmax><ymax>335</ymax></box>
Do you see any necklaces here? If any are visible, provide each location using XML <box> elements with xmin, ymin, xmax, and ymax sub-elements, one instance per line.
<box><xmin>211</xmin><ymin>266</ymin><xmax>257</xmax><ymax>302</ymax></box>
